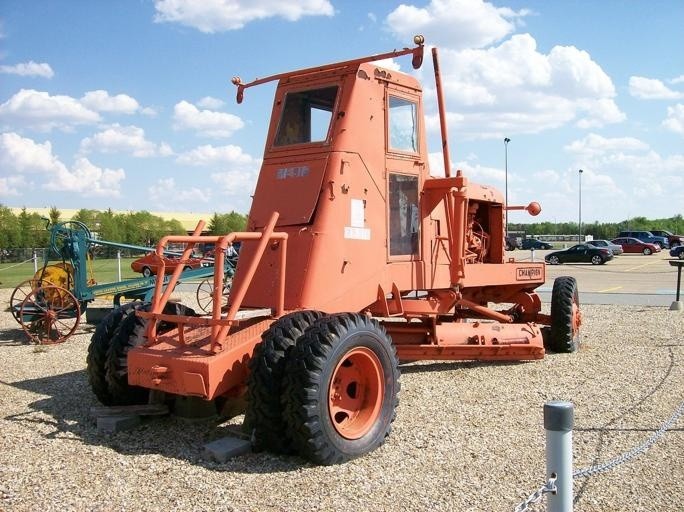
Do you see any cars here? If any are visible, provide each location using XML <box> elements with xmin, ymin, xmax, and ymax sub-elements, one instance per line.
<box><xmin>545</xmin><ymin>230</ymin><xmax>684</xmax><ymax>265</ymax></box>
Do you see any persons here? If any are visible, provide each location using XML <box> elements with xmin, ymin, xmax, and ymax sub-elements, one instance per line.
<box><xmin>224</xmin><ymin>242</ymin><xmax>238</xmax><ymax>264</ymax></box>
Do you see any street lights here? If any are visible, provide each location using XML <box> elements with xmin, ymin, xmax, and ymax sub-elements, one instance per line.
<box><xmin>578</xmin><ymin>169</ymin><xmax>583</xmax><ymax>244</ymax></box>
